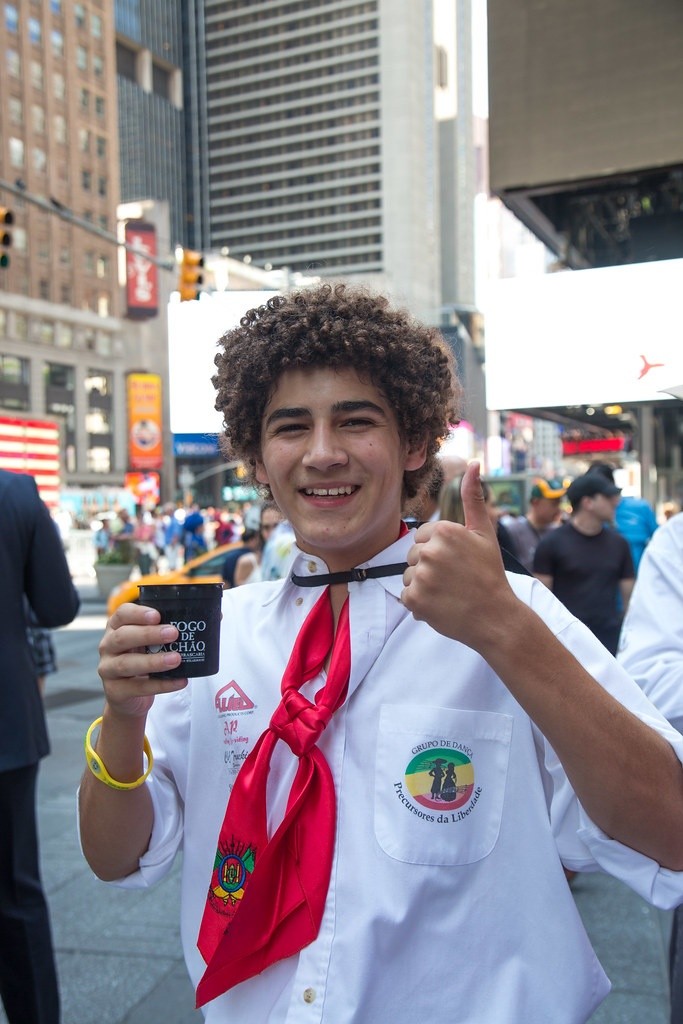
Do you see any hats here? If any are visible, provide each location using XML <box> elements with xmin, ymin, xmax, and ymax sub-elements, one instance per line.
<box><xmin>531</xmin><ymin>477</ymin><xmax>570</xmax><ymax>499</ymax></box>
<box><xmin>567</xmin><ymin>472</ymin><xmax>623</xmax><ymax>503</ymax></box>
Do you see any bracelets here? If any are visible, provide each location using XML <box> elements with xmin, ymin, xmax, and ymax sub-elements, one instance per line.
<box><xmin>85</xmin><ymin>716</ymin><xmax>153</xmax><ymax>789</ymax></box>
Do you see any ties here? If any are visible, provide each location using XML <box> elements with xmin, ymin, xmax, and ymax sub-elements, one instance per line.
<box><xmin>195</xmin><ymin>517</ymin><xmax>410</xmax><ymax>1008</ymax></box>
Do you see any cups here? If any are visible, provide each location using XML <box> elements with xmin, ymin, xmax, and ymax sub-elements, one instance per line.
<box><xmin>138</xmin><ymin>583</ymin><xmax>223</xmax><ymax>680</ymax></box>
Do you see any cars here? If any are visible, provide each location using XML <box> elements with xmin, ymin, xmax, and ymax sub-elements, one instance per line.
<box><xmin>103</xmin><ymin>539</ymin><xmax>244</xmax><ymax>620</ymax></box>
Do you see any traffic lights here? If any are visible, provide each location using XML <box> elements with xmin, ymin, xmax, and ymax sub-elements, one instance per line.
<box><xmin>176</xmin><ymin>248</ymin><xmax>206</xmax><ymax>303</ymax></box>
<box><xmin>0</xmin><ymin>205</ymin><xmax>16</xmax><ymax>271</ymax></box>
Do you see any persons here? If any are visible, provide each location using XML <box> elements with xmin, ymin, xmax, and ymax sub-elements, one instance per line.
<box><xmin>0</xmin><ymin>469</ymin><xmax>83</xmax><ymax>1024</ymax></box>
<box><xmin>403</xmin><ymin>457</ymin><xmax>683</xmax><ymax>658</ymax></box>
<box><xmin>95</xmin><ymin>493</ymin><xmax>289</xmax><ymax>589</ymax></box>
<box><xmin>78</xmin><ymin>281</ymin><xmax>683</xmax><ymax>1024</ymax></box>
<box><xmin>615</xmin><ymin>511</ymin><xmax>683</xmax><ymax>1024</ymax></box>
